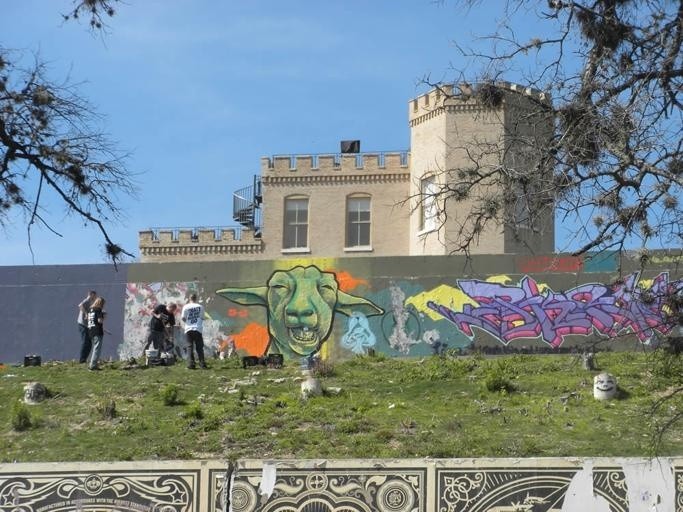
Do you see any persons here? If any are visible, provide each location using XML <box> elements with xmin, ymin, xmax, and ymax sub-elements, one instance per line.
<box><xmin>88</xmin><ymin>296</ymin><xmax>106</xmax><ymax>370</ymax></box>
<box><xmin>142</xmin><ymin>303</ymin><xmax>179</xmax><ymax>355</ymax></box>
<box><xmin>77</xmin><ymin>290</ymin><xmax>99</xmax><ymax>363</ymax></box>
<box><xmin>182</xmin><ymin>293</ymin><xmax>211</xmax><ymax>370</ymax></box>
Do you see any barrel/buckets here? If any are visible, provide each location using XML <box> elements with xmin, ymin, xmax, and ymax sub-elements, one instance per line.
<box><xmin>145</xmin><ymin>350</ymin><xmax>159</xmax><ymax>364</ymax></box>
